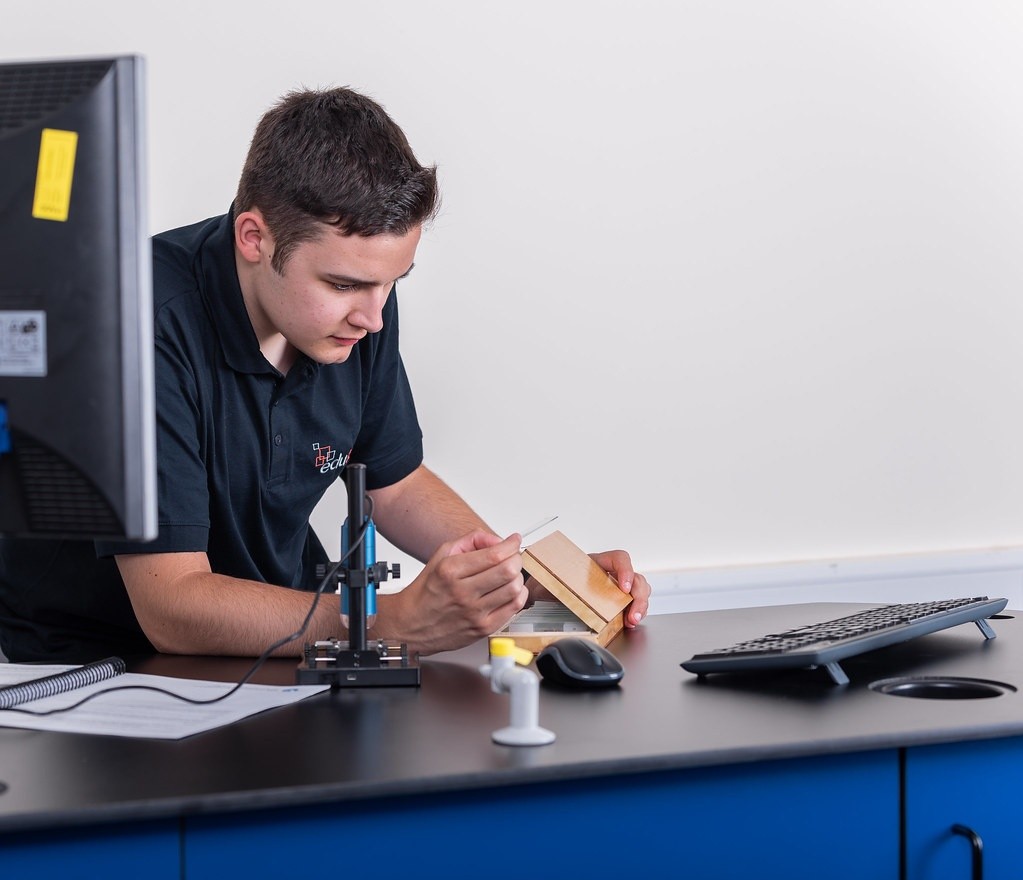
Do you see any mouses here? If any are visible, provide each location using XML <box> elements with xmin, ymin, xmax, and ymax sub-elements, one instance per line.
<box><xmin>535</xmin><ymin>637</ymin><xmax>625</xmax><ymax>686</ymax></box>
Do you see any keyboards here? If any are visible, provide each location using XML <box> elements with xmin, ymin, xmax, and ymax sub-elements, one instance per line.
<box><xmin>680</xmin><ymin>596</ymin><xmax>1008</xmax><ymax>695</ymax></box>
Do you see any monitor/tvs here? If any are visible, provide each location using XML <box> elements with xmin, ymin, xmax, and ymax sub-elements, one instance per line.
<box><xmin>0</xmin><ymin>53</ymin><xmax>158</xmax><ymax>545</ymax></box>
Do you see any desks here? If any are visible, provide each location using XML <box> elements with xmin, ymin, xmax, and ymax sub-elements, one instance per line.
<box><xmin>0</xmin><ymin>600</ymin><xmax>1023</xmax><ymax>880</ymax></box>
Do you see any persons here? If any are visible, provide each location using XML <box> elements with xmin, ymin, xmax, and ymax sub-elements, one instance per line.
<box><xmin>0</xmin><ymin>90</ymin><xmax>654</xmax><ymax>661</ymax></box>
